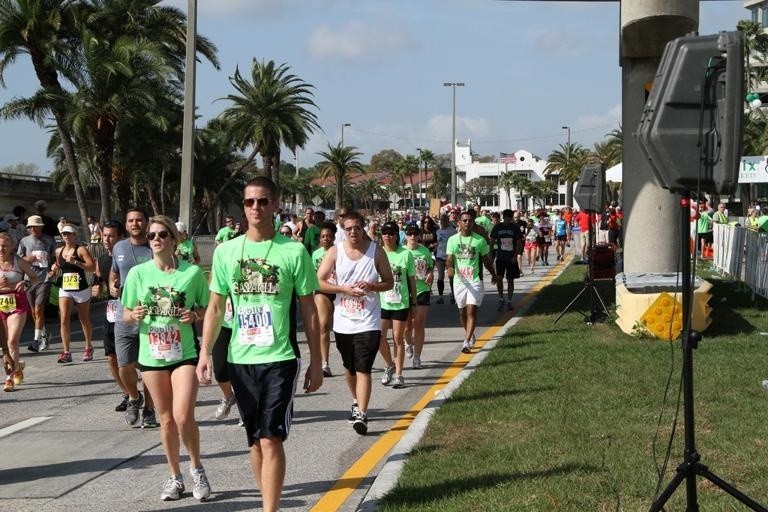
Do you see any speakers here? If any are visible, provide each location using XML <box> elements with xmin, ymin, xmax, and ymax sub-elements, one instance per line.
<box><xmin>632</xmin><ymin>29</ymin><xmax>744</xmax><ymax>195</ymax></box>
<box><xmin>573</xmin><ymin>165</ymin><xmax>606</xmax><ymax>214</ymax></box>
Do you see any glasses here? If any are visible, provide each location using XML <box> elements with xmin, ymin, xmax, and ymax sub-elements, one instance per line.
<box><xmin>146</xmin><ymin>231</ymin><xmax>171</xmax><ymax>239</ymax></box>
<box><xmin>382</xmin><ymin>230</ymin><xmax>397</xmax><ymax>236</ymax></box>
<box><xmin>104</xmin><ymin>221</ymin><xmax>120</xmax><ymax>226</ymax></box>
<box><xmin>405</xmin><ymin>231</ymin><xmax>420</xmax><ymax>236</ymax></box>
<box><xmin>280</xmin><ymin>233</ymin><xmax>292</xmax><ymax>235</ymax></box>
<box><xmin>345</xmin><ymin>226</ymin><xmax>361</xmax><ymax>231</ymax></box>
<box><xmin>460</xmin><ymin>219</ymin><xmax>473</xmax><ymax>223</ymax></box>
<box><xmin>243</xmin><ymin>198</ymin><xmax>270</xmax><ymax>207</ymax></box>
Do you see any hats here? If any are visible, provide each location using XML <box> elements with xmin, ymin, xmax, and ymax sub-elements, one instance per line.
<box><xmin>175</xmin><ymin>222</ymin><xmax>186</xmax><ymax>234</ymax></box>
<box><xmin>0</xmin><ymin>200</ymin><xmax>48</xmax><ymax>230</ymax></box>
<box><xmin>381</xmin><ymin>223</ymin><xmax>399</xmax><ymax>231</ymax></box>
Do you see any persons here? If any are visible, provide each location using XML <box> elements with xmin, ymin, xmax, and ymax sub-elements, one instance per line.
<box><xmin>377</xmin><ymin>221</ymin><xmax>419</xmax><ymax>388</ymax></box>
<box><xmin>316</xmin><ymin>211</ymin><xmax>393</xmax><ymax>436</ymax></box>
<box><xmin>697</xmin><ymin>200</ymin><xmax>729</xmax><ymax>258</ymax></box>
<box><xmin>274</xmin><ymin>207</ymin><xmax>325</xmax><ymax>255</ymax></box>
<box><xmin>401</xmin><ymin>223</ymin><xmax>434</xmax><ymax>368</ymax></box>
<box><xmin>91</xmin><ymin>219</ymin><xmax>146</xmax><ymax>410</ymax></box>
<box><xmin>333</xmin><ymin>208</ymin><xmax>347</xmax><ymax>245</ymax></box>
<box><xmin>520</xmin><ymin>207</ymin><xmax>573</xmax><ymax>275</ymax></box>
<box><xmin>312</xmin><ymin>222</ymin><xmax>337</xmax><ymax>377</ymax></box>
<box><xmin>175</xmin><ymin>222</ymin><xmax>201</xmax><ymax>265</ymax></box>
<box><xmin>690</xmin><ymin>219</ymin><xmax>697</xmax><ymax>259</ymax></box>
<box><xmin>364</xmin><ymin>208</ymin><xmax>440</xmax><ymax>295</ymax></box>
<box><xmin>435</xmin><ymin>205</ymin><xmax>523</xmax><ymax>354</ymax></box>
<box><xmin>746</xmin><ymin>200</ymin><xmax>768</xmax><ymax>233</ymax></box>
<box><xmin>572</xmin><ymin>206</ymin><xmax>624</xmax><ymax>261</ymax></box>
<box><xmin>106</xmin><ymin>206</ymin><xmax>164</xmax><ymax>429</ymax></box>
<box><xmin>122</xmin><ymin>214</ymin><xmax>213</xmax><ymax>502</ymax></box>
<box><xmin>215</xmin><ymin>214</ymin><xmax>249</xmax><ymax>244</ymax></box>
<box><xmin>194</xmin><ymin>177</ymin><xmax>322</xmax><ymax>511</ymax></box>
<box><xmin>207</xmin><ymin>231</ymin><xmax>245</xmax><ymax>428</ymax></box>
<box><xmin>1</xmin><ymin>199</ymin><xmax>103</xmax><ymax>392</ymax></box>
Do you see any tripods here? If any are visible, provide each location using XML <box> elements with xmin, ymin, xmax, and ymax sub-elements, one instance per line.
<box><xmin>649</xmin><ymin>195</ymin><xmax>768</xmax><ymax>512</ymax></box>
<box><xmin>552</xmin><ymin>214</ymin><xmax>610</xmax><ymax>324</ymax></box>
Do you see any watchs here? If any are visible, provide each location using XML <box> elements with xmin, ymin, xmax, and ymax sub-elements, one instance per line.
<box><xmin>412</xmin><ymin>303</ymin><xmax>417</xmax><ymax>307</ymax></box>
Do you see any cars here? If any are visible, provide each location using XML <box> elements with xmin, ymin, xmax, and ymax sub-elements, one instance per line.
<box><xmin>324</xmin><ymin>210</ymin><xmax>336</xmax><ymax>221</ymax></box>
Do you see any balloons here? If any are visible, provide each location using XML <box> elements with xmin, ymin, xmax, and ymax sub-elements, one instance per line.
<box><xmin>691</xmin><ymin>199</ymin><xmax>697</xmax><ymax>217</ymax></box>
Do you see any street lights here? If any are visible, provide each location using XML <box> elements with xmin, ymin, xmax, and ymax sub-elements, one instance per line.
<box><xmin>416</xmin><ymin>148</ymin><xmax>422</xmax><ymax>206</ymax></box>
<box><xmin>341</xmin><ymin>123</ymin><xmax>351</xmax><ymax>150</ymax></box>
<box><xmin>444</xmin><ymin>83</ymin><xmax>466</xmax><ymax>206</ymax></box>
<box><xmin>562</xmin><ymin>126</ymin><xmax>571</xmax><ymax>167</ymax></box>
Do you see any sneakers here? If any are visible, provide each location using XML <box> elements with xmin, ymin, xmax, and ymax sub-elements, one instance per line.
<box><xmin>189</xmin><ymin>465</ymin><xmax>211</xmax><ymax>501</ymax></box>
<box><xmin>28</xmin><ymin>340</ymin><xmax>42</xmax><ymax>353</ymax></box>
<box><xmin>83</xmin><ymin>345</ymin><xmax>94</xmax><ymax>361</ymax></box>
<box><xmin>449</xmin><ymin>293</ymin><xmax>454</xmax><ymax>304</ymax></box>
<box><xmin>405</xmin><ymin>343</ymin><xmax>413</xmax><ymax>359</ymax></box>
<box><xmin>321</xmin><ymin>363</ymin><xmax>332</xmax><ymax>377</ymax></box>
<box><xmin>393</xmin><ymin>375</ymin><xmax>404</xmax><ymax>389</ymax></box>
<box><xmin>216</xmin><ymin>393</ymin><xmax>236</xmax><ymax>418</ymax></box>
<box><xmin>519</xmin><ymin>255</ymin><xmax>564</xmax><ymax>276</ymax></box>
<box><xmin>40</xmin><ymin>330</ymin><xmax>51</xmax><ymax>350</ymax></box>
<box><xmin>462</xmin><ymin>339</ymin><xmax>471</xmax><ymax>352</ymax></box>
<box><xmin>3</xmin><ymin>379</ymin><xmax>14</xmax><ymax>392</ymax></box>
<box><xmin>505</xmin><ymin>302</ymin><xmax>512</xmax><ymax>311</ymax></box>
<box><xmin>436</xmin><ymin>295</ymin><xmax>444</xmax><ymax>304</ymax></box>
<box><xmin>382</xmin><ymin>362</ymin><xmax>396</xmax><ymax>385</ymax></box>
<box><xmin>161</xmin><ymin>475</ymin><xmax>185</xmax><ymax>501</ymax></box>
<box><xmin>353</xmin><ymin>411</ymin><xmax>367</xmax><ymax>435</ymax></box>
<box><xmin>349</xmin><ymin>403</ymin><xmax>358</xmax><ymax>423</ymax></box>
<box><xmin>468</xmin><ymin>335</ymin><xmax>476</xmax><ymax>347</ymax></box>
<box><xmin>115</xmin><ymin>390</ymin><xmax>157</xmax><ymax>428</ymax></box>
<box><xmin>495</xmin><ymin>298</ymin><xmax>505</xmax><ymax>311</ymax></box>
<box><xmin>57</xmin><ymin>353</ymin><xmax>72</xmax><ymax>363</ymax></box>
<box><xmin>13</xmin><ymin>361</ymin><xmax>25</xmax><ymax>385</ymax></box>
<box><xmin>413</xmin><ymin>356</ymin><xmax>421</xmax><ymax>368</ymax></box>
